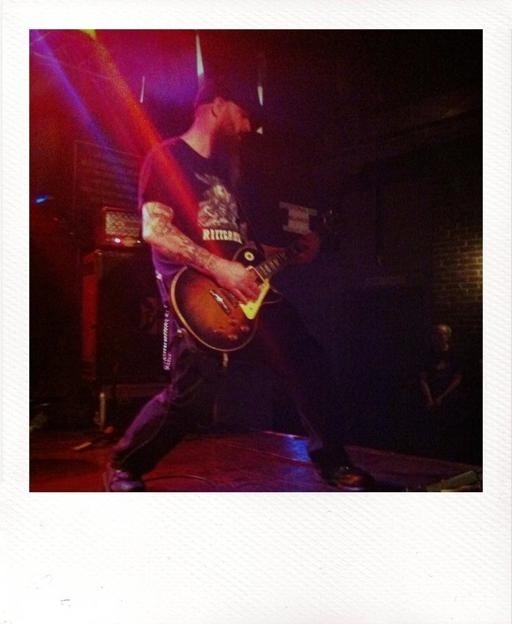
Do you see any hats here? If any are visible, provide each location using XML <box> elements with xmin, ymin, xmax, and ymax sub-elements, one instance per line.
<box><xmin>195</xmin><ymin>75</ymin><xmax>265</xmax><ymax>123</ymax></box>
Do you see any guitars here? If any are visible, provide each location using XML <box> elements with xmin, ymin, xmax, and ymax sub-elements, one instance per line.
<box><xmin>168</xmin><ymin>207</ymin><xmax>343</xmax><ymax>353</ymax></box>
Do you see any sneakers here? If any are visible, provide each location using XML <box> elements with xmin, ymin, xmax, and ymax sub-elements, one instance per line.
<box><xmin>321</xmin><ymin>462</ymin><xmax>376</xmax><ymax>489</ymax></box>
<box><xmin>108</xmin><ymin>461</ymin><xmax>144</xmax><ymax>491</ymax></box>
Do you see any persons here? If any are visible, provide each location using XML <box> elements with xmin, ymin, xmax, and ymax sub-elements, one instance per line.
<box><xmin>104</xmin><ymin>72</ymin><xmax>380</xmax><ymax>492</ymax></box>
<box><xmin>417</xmin><ymin>320</ymin><xmax>462</xmax><ymax>411</ymax></box>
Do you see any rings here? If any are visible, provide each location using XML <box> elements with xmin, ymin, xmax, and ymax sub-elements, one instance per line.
<box><xmin>251</xmin><ymin>281</ymin><xmax>258</xmax><ymax>291</ymax></box>
